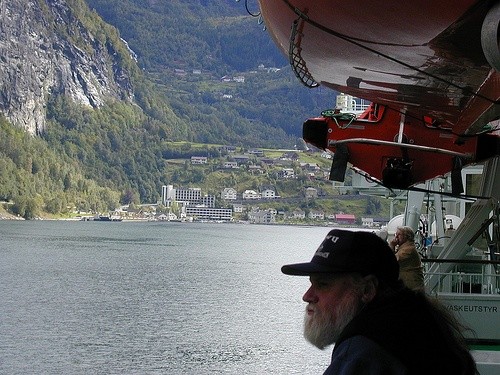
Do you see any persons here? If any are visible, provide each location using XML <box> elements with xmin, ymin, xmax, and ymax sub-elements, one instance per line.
<box><xmin>390</xmin><ymin>227</ymin><xmax>426</xmax><ymax>293</ymax></box>
<box><xmin>281</xmin><ymin>229</ymin><xmax>482</xmax><ymax>375</ymax></box>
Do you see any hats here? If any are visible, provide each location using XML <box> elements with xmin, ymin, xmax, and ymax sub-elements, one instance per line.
<box><xmin>281</xmin><ymin>229</ymin><xmax>400</xmax><ymax>280</ymax></box>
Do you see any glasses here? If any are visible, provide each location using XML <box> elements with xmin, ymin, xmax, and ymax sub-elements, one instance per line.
<box><xmin>394</xmin><ymin>232</ymin><xmax>404</xmax><ymax>237</ymax></box>
